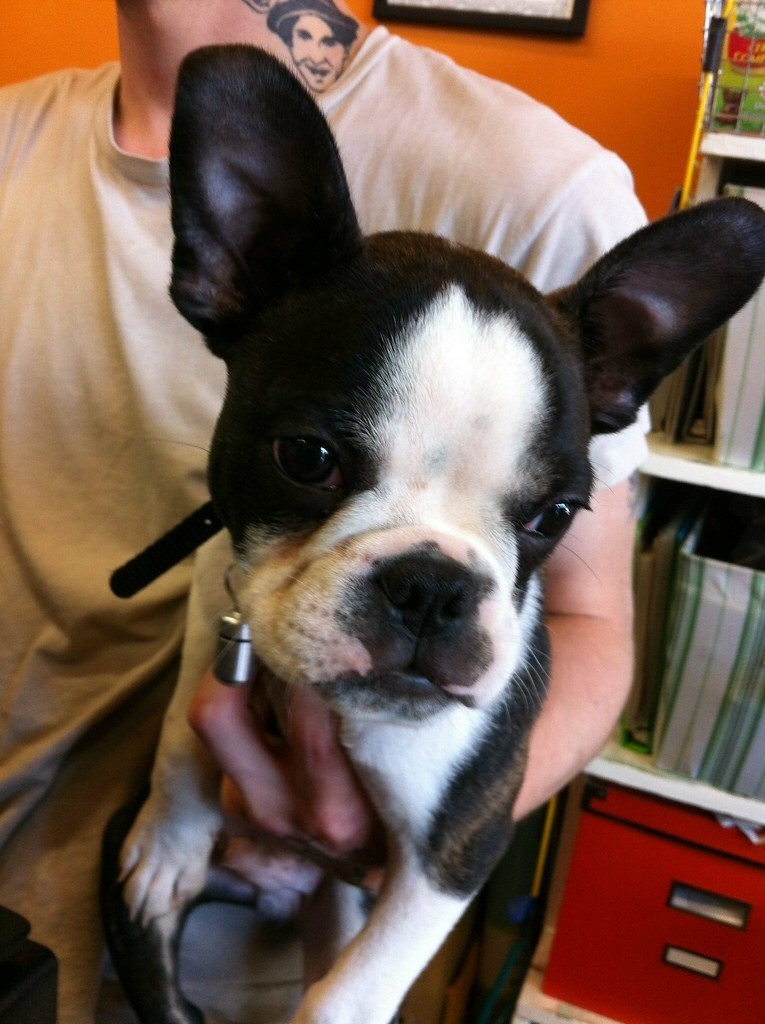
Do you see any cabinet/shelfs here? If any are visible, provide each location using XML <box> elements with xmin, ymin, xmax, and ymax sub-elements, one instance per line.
<box><xmin>484</xmin><ymin>0</ymin><xmax>765</xmax><ymax>1024</ymax></box>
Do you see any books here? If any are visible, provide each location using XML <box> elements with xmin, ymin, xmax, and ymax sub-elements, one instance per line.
<box><xmin>625</xmin><ymin>183</ymin><xmax>765</xmax><ymax>805</ymax></box>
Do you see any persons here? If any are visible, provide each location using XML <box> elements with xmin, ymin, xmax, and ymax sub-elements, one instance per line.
<box><xmin>0</xmin><ymin>0</ymin><xmax>650</xmax><ymax>1024</ymax></box>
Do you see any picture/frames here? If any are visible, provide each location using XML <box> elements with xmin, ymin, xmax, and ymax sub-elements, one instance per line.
<box><xmin>373</xmin><ymin>0</ymin><xmax>590</xmax><ymax>41</ymax></box>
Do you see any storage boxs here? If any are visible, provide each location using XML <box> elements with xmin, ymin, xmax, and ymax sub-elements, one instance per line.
<box><xmin>540</xmin><ymin>776</ymin><xmax>764</xmax><ymax>1024</ymax></box>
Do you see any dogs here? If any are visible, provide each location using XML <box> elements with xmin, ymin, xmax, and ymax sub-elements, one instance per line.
<box><xmin>94</xmin><ymin>42</ymin><xmax>765</xmax><ymax>1024</ymax></box>
